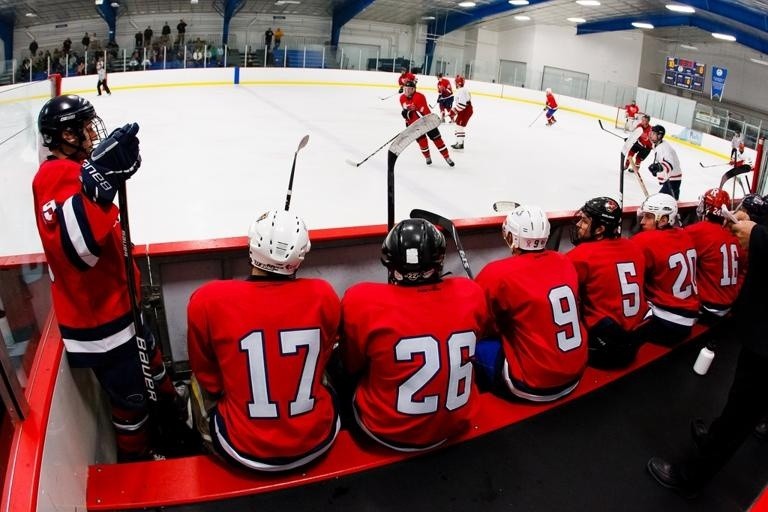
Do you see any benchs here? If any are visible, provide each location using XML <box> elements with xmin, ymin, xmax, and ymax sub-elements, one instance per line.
<box><xmin>86</xmin><ymin>321</ymin><xmax>710</xmax><ymax>511</ymax></box>
<box><xmin>743</xmin><ymin>485</ymin><xmax>768</xmax><ymax>512</ymax></box>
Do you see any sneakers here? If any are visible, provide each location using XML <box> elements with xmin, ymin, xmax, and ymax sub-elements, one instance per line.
<box><xmin>426</xmin><ymin>158</ymin><xmax>433</xmax><ymax>165</ymax></box>
<box><xmin>446</xmin><ymin>158</ymin><xmax>455</xmax><ymax>167</ymax></box>
<box><xmin>451</xmin><ymin>142</ymin><xmax>458</xmax><ymax>148</ymax></box>
<box><xmin>454</xmin><ymin>143</ymin><xmax>464</xmax><ymax>149</ymax></box>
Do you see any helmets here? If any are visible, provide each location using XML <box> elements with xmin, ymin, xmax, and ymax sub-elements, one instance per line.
<box><xmin>400</xmin><ymin>66</ymin><xmax>465</xmax><ymax>91</ymax></box>
<box><xmin>739</xmin><ymin>144</ymin><xmax>744</xmax><ymax>152</ymax></box>
<box><xmin>380</xmin><ymin>217</ymin><xmax>452</xmax><ymax>285</ymax></box>
<box><xmin>546</xmin><ymin>88</ymin><xmax>552</xmax><ymax>94</ymax></box>
<box><xmin>636</xmin><ymin>193</ymin><xmax>678</xmax><ymax>230</ymax></box>
<box><xmin>695</xmin><ymin>188</ymin><xmax>731</xmax><ymax>222</ymax></box>
<box><xmin>569</xmin><ymin>195</ymin><xmax>623</xmax><ymax>246</ymax></box>
<box><xmin>500</xmin><ymin>205</ymin><xmax>552</xmax><ymax>252</ymax></box>
<box><xmin>248</xmin><ymin>210</ymin><xmax>312</xmax><ymax>276</ymax></box>
<box><xmin>38</xmin><ymin>92</ymin><xmax>109</xmax><ymax>159</ymax></box>
<box><xmin>742</xmin><ymin>194</ymin><xmax>767</xmax><ymax>221</ymax></box>
<box><xmin>628</xmin><ymin>100</ymin><xmax>665</xmax><ymax>143</ymax></box>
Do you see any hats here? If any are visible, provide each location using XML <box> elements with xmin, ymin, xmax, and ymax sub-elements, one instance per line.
<box><xmin>735</xmin><ymin>130</ymin><xmax>740</xmax><ymax>137</ymax></box>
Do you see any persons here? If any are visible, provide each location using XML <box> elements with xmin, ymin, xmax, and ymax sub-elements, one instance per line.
<box><xmin>329</xmin><ymin>218</ymin><xmax>490</xmax><ymax>456</ymax></box>
<box><xmin>542</xmin><ymin>87</ymin><xmax>560</xmax><ymax>127</ymax></box>
<box><xmin>472</xmin><ymin>202</ymin><xmax>589</xmax><ymax>405</ymax></box>
<box><xmin>30</xmin><ymin>92</ymin><xmax>190</xmax><ymax>463</ymax></box>
<box><xmin>272</xmin><ymin>27</ymin><xmax>284</xmax><ymax>50</ymax></box>
<box><xmin>621</xmin><ymin>100</ymin><xmax>767</xmax><ymax>347</ymax></box>
<box><xmin>265</xmin><ymin>27</ymin><xmax>274</xmax><ymax>49</ymax></box>
<box><xmin>16</xmin><ymin>19</ymin><xmax>224</xmax><ymax>85</ymax></box>
<box><xmin>566</xmin><ymin>196</ymin><xmax>653</xmax><ymax>372</ymax></box>
<box><xmin>395</xmin><ymin>66</ymin><xmax>474</xmax><ymax>167</ymax></box>
<box><xmin>185</xmin><ymin>207</ymin><xmax>343</xmax><ymax>478</ymax></box>
<box><xmin>644</xmin><ymin>210</ymin><xmax>768</xmax><ymax>502</ymax></box>
<box><xmin>96</xmin><ymin>62</ymin><xmax>111</xmax><ymax>97</ymax></box>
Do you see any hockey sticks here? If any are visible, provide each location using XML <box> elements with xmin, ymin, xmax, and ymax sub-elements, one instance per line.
<box><xmin>345</xmin><ymin>132</ymin><xmax>401</xmax><ymax>167</ymax></box>
<box><xmin>719</xmin><ymin>165</ymin><xmax>750</xmax><ymax>189</ymax></box>
<box><xmin>493</xmin><ymin>201</ymin><xmax>520</xmax><ymax>210</ymax></box>
<box><xmin>700</xmin><ymin>162</ymin><xmax>731</xmax><ymax>167</ymax></box>
<box><xmin>285</xmin><ymin>135</ymin><xmax>309</xmax><ymax>211</ymax></box>
<box><xmin>734</xmin><ymin>176</ymin><xmax>746</xmax><ymax>195</ymax></box>
<box><xmin>410</xmin><ymin>209</ymin><xmax>473</xmax><ymax>280</ymax></box>
<box><xmin>388</xmin><ymin>114</ymin><xmax>441</xmax><ymax>232</ymax></box>
<box><xmin>599</xmin><ymin>120</ymin><xmax>624</xmax><ymax>139</ymax></box>
<box><xmin>617</xmin><ymin>127</ymin><xmax>643</xmax><ymax>238</ymax></box>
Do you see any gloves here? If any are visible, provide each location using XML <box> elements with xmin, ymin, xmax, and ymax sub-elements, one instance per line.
<box><xmin>729</xmin><ymin>161</ymin><xmax>734</xmax><ymax>165</ymax></box>
<box><xmin>649</xmin><ymin>163</ymin><xmax>662</xmax><ymax>176</ymax></box>
<box><xmin>399</xmin><ymin>89</ymin><xmax>403</xmax><ymax>93</ymax></box>
<box><xmin>449</xmin><ymin>109</ymin><xmax>458</xmax><ymax>119</ymax></box>
<box><xmin>401</xmin><ymin>109</ymin><xmax>412</xmax><ymax>120</ymax></box>
<box><xmin>82</xmin><ymin>121</ymin><xmax>142</xmax><ymax>211</ymax></box>
